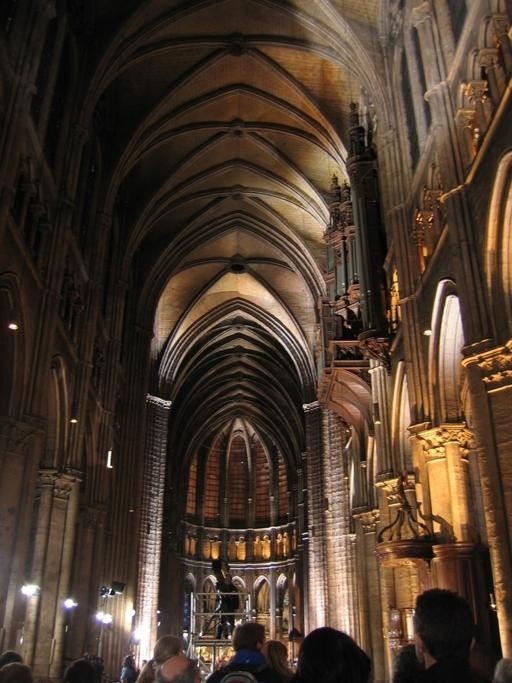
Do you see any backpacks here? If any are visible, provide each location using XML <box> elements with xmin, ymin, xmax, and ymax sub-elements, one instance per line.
<box><xmin>219</xmin><ymin>663</ymin><xmax>266</xmax><ymax>683</ymax></box>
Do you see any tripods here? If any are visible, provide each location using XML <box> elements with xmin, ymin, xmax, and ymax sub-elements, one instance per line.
<box><xmin>202</xmin><ymin>598</ymin><xmax>235</xmax><ymax>638</ymax></box>
